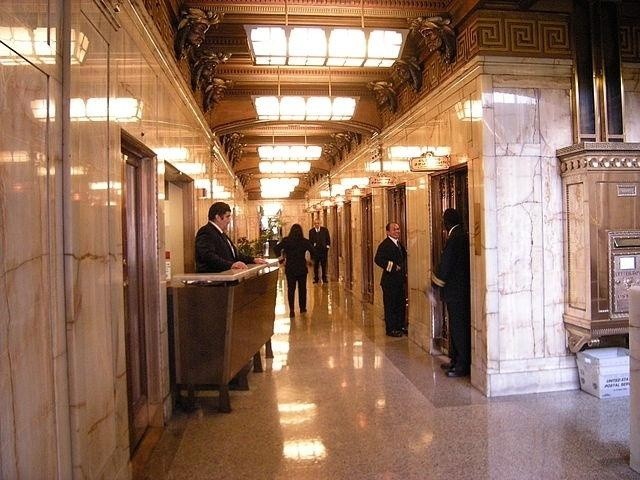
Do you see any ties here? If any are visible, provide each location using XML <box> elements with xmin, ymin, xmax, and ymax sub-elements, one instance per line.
<box><xmin>398</xmin><ymin>240</ymin><xmax>403</xmax><ymax>258</ymax></box>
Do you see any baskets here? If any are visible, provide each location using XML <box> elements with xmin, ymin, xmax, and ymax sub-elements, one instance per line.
<box><xmin>576</xmin><ymin>347</ymin><xmax>630</xmax><ymax>399</ymax></box>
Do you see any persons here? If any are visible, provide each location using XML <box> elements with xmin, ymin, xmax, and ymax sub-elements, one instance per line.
<box><xmin>195</xmin><ymin>202</ymin><xmax>268</xmax><ymax>273</ymax></box>
<box><xmin>274</xmin><ymin>224</ymin><xmax>318</xmax><ymax>317</ymax></box>
<box><xmin>431</xmin><ymin>208</ymin><xmax>471</xmax><ymax>377</ymax></box>
<box><xmin>309</xmin><ymin>218</ymin><xmax>330</xmax><ymax>283</ymax></box>
<box><xmin>374</xmin><ymin>222</ymin><xmax>407</xmax><ymax>337</ymax></box>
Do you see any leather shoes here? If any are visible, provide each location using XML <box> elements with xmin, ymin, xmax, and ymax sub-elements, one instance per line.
<box><xmin>313</xmin><ymin>280</ymin><xmax>328</xmax><ymax>283</ymax></box>
<box><xmin>290</xmin><ymin>309</ymin><xmax>307</xmax><ymax>317</ymax></box>
<box><xmin>441</xmin><ymin>363</ymin><xmax>467</xmax><ymax>377</ymax></box>
<box><xmin>386</xmin><ymin>328</ymin><xmax>403</xmax><ymax>337</ymax></box>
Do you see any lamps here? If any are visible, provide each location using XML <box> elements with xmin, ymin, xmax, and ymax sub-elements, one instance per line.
<box><xmin>244</xmin><ymin>24</ymin><xmax>410</xmax><ymax>70</ymax></box>
<box><xmin>256</xmin><ymin>144</ymin><xmax>323</xmax><ymax>161</ymax></box>
<box><xmin>260</xmin><ymin>177</ymin><xmax>299</xmax><ymax>198</ymax></box>
<box><xmin>258</xmin><ymin>162</ymin><xmax>311</xmax><ymax>174</ymax></box>
<box><xmin>251</xmin><ymin>94</ymin><xmax>360</xmax><ymax>122</ymax></box>
